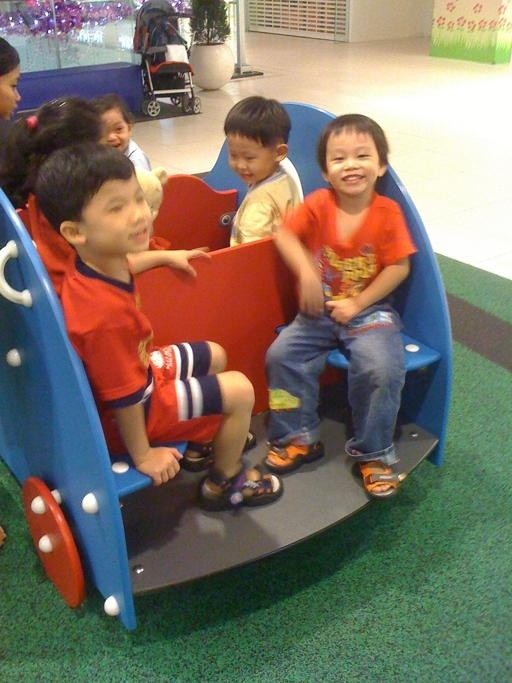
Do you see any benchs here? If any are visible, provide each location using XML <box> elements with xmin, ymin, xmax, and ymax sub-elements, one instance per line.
<box><xmin>15</xmin><ymin>60</ymin><xmax>146</xmax><ymax>119</ymax></box>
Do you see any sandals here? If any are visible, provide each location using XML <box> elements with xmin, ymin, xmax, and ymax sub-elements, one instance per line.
<box><xmin>179</xmin><ymin>424</ymin><xmax>257</xmax><ymax>474</ymax></box>
<box><xmin>262</xmin><ymin>434</ymin><xmax>326</xmax><ymax>476</ymax></box>
<box><xmin>357</xmin><ymin>457</ymin><xmax>401</xmax><ymax>501</ymax></box>
<box><xmin>196</xmin><ymin>458</ymin><xmax>287</xmax><ymax>512</ymax></box>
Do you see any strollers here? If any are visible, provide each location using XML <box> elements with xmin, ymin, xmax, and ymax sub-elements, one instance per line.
<box><xmin>131</xmin><ymin>0</ymin><xmax>201</xmax><ymax>117</ymax></box>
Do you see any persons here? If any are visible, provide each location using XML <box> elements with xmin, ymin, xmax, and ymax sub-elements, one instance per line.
<box><xmin>220</xmin><ymin>97</ymin><xmax>306</xmax><ymax>246</ymax></box>
<box><xmin>2</xmin><ymin>96</ymin><xmax>107</xmax><ymax>295</ymax></box>
<box><xmin>32</xmin><ymin>142</ymin><xmax>285</xmax><ymax>509</ymax></box>
<box><xmin>273</xmin><ymin>113</ymin><xmax>415</xmax><ymax>499</ymax></box>
<box><xmin>1</xmin><ymin>36</ymin><xmax>22</xmax><ymax>120</ymax></box>
<box><xmin>94</xmin><ymin>95</ymin><xmax>152</xmax><ymax>178</ymax></box>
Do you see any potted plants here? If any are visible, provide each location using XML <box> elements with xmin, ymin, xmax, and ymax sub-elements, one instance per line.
<box><xmin>189</xmin><ymin>0</ymin><xmax>236</xmax><ymax>90</ymax></box>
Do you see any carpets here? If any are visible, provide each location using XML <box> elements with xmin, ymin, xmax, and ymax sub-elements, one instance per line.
<box><xmin>132</xmin><ymin>99</ymin><xmax>204</xmax><ymax>123</ymax></box>
